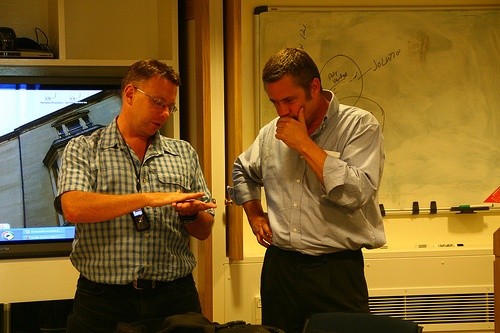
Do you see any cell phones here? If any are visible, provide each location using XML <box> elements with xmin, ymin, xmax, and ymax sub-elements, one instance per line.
<box><xmin>130</xmin><ymin>208</ymin><xmax>150</xmax><ymax>232</ymax></box>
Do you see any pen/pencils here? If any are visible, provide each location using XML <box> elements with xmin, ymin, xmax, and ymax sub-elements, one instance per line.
<box><xmin>459</xmin><ymin>203</ymin><xmax>495</xmax><ymax>209</ymax></box>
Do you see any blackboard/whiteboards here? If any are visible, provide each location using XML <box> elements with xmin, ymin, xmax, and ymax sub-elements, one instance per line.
<box><xmin>252</xmin><ymin>5</ymin><xmax>500</xmax><ymax>213</ymax></box>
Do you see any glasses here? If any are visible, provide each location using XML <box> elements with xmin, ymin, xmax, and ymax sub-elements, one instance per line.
<box><xmin>135</xmin><ymin>87</ymin><xmax>177</xmax><ymax>115</ymax></box>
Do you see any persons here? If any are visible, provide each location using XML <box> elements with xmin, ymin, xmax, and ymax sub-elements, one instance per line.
<box><xmin>227</xmin><ymin>48</ymin><xmax>387</xmax><ymax>333</ymax></box>
<box><xmin>54</xmin><ymin>59</ymin><xmax>216</xmax><ymax>333</ymax></box>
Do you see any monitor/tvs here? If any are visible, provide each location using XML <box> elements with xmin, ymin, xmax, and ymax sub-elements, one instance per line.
<box><xmin>0</xmin><ymin>65</ymin><xmax>130</xmax><ymax>260</ymax></box>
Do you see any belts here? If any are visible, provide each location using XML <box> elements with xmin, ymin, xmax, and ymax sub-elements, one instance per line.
<box><xmin>80</xmin><ymin>272</ymin><xmax>194</xmax><ymax>290</ymax></box>
<box><xmin>268</xmin><ymin>245</ymin><xmax>362</xmax><ymax>264</ymax></box>
